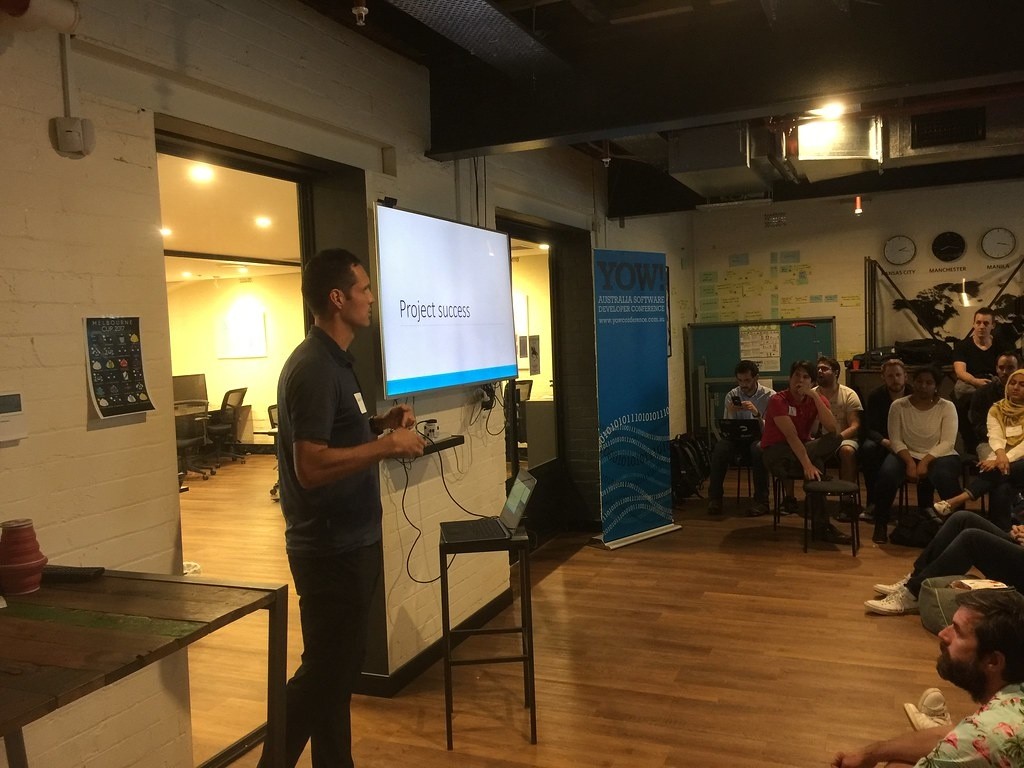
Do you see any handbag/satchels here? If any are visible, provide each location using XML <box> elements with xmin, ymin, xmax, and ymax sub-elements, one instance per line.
<box><xmin>890</xmin><ymin>509</ymin><xmax>941</xmax><ymax>548</ymax></box>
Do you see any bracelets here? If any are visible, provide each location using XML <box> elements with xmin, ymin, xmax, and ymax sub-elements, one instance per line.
<box><xmin>368</xmin><ymin>415</ymin><xmax>384</xmax><ymax>435</ymax></box>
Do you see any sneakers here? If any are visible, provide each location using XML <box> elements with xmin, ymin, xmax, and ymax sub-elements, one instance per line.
<box><xmin>836</xmin><ymin>501</ymin><xmax>854</xmax><ymax>523</ymax></box>
<box><xmin>863</xmin><ymin>583</ymin><xmax>919</xmax><ymax>615</ymax></box>
<box><xmin>778</xmin><ymin>495</ymin><xmax>799</xmax><ymax>515</ymax></box>
<box><xmin>745</xmin><ymin>496</ymin><xmax>770</xmax><ymax>516</ymax></box>
<box><xmin>709</xmin><ymin>499</ymin><xmax>724</xmax><ymax>516</ymax></box>
<box><xmin>918</xmin><ymin>687</ymin><xmax>951</xmax><ymax>726</ymax></box>
<box><xmin>873</xmin><ymin>572</ymin><xmax>913</xmax><ymax>594</ymax></box>
<box><xmin>903</xmin><ymin>701</ymin><xmax>942</xmax><ymax>730</ymax></box>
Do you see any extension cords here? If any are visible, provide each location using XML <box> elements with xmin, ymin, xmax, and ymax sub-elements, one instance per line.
<box><xmin>482</xmin><ymin>388</ymin><xmax>497</xmax><ymax>409</ymax></box>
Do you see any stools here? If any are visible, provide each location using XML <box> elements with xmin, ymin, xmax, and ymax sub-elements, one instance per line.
<box><xmin>736</xmin><ymin>459</ymin><xmax>984</xmax><ymax>558</ymax></box>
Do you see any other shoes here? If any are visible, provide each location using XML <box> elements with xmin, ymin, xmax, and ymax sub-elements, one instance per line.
<box><xmin>928</xmin><ymin>508</ymin><xmax>945</xmax><ymax>525</ymax></box>
<box><xmin>859</xmin><ymin>505</ymin><xmax>875</xmax><ymax>519</ymax></box>
<box><xmin>872</xmin><ymin>519</ymin><xmax>887</xmax><ymax>543</ymax></box>
<box><xmin>934</xmin><ymin>500</ymin><xmax>954</xmax><ymax>517</ymax></box>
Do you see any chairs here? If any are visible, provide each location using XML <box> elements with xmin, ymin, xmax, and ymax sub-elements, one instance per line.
<box><xmin>268</xmin><ymin>404</ymin><xmax>280</xmax><ymax>495</ymax></box>
<box><xmin>174</xmin><ymin>388</ymin><xmax>247</xmax><ymax>480</ymax></box>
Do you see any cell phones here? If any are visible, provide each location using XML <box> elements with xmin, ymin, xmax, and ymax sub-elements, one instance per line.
<box><xmin>732</xmin><ymin>395</ymin><xmax>742</xmax><ymax>407</ymax></box>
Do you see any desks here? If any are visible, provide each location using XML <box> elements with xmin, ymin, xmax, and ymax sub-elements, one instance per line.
<box><xmin>0</xmin><ymin>564</ymin><xmax>288</xmax><ymax>768</ymax></box>
<box><xmin>174</xmin><ymin>404</ymin><xmax>251</xmax><ymax>461</ymax></box>
<box><xmin>438</xmin><ymin>522</ymin><xmax>538</xmax><ymax>751</ymax></box>
<box><xmin>251</xmin><ymin>427</ymin><xmax>278</xmax><ymax>456</ymax></box>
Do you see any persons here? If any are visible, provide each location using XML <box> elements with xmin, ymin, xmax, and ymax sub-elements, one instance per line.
<box><xmin>763</xmin><ymin>360</ymin><xmax>854</xmax><ymax>543</ymax></box>
<box><xmin>256</xmin><ymin>251</ymin><xmax>428</xmax><ymax>768</ymax></box>
<box><xmin>829</xmin><ymin>308</ymin><xmax>1024</xmax><ymax>768</ymax></box>
<box><xmin>704</xmin><ymin>360</ymin><xmax>775</xmax><ymax>517</ymax></box>
<box><xmin>808</xmin><ymin>355</ymin><xmax>863</xmax><ymax>520</ymax></box>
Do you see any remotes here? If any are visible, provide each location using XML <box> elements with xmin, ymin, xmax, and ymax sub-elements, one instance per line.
<box><xmin>42</xmin><ymin>566</ymin><xmax>106</xmax><ymax>585</ymax></box>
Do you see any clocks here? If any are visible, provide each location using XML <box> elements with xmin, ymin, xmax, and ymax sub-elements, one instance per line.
<box><xmin>883</xmin><ymin>235</ymin><xmax>917</xmax><ymax>266</ymax></box>
<box><xmin>932</xmin><ymin>231</ymin><xmax>967</xmax><ymax>262</ymax></box>
<box><xmin>981</xmin><ymin>228</ymin><xmax>1016</xmax><ymax>259</ymax></box>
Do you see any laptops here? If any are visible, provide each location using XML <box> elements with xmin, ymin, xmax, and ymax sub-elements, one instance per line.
<box><xmin>440</xmin><ymin>467</ymin><xmax>537</xmax><ymax>543</ymax></box>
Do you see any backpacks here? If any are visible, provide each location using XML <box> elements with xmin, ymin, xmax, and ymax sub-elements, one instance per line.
<box><xmin>670</xmin><ymin>433</ymin><xmax>712</xmax><ymax>498</ymax></box>
